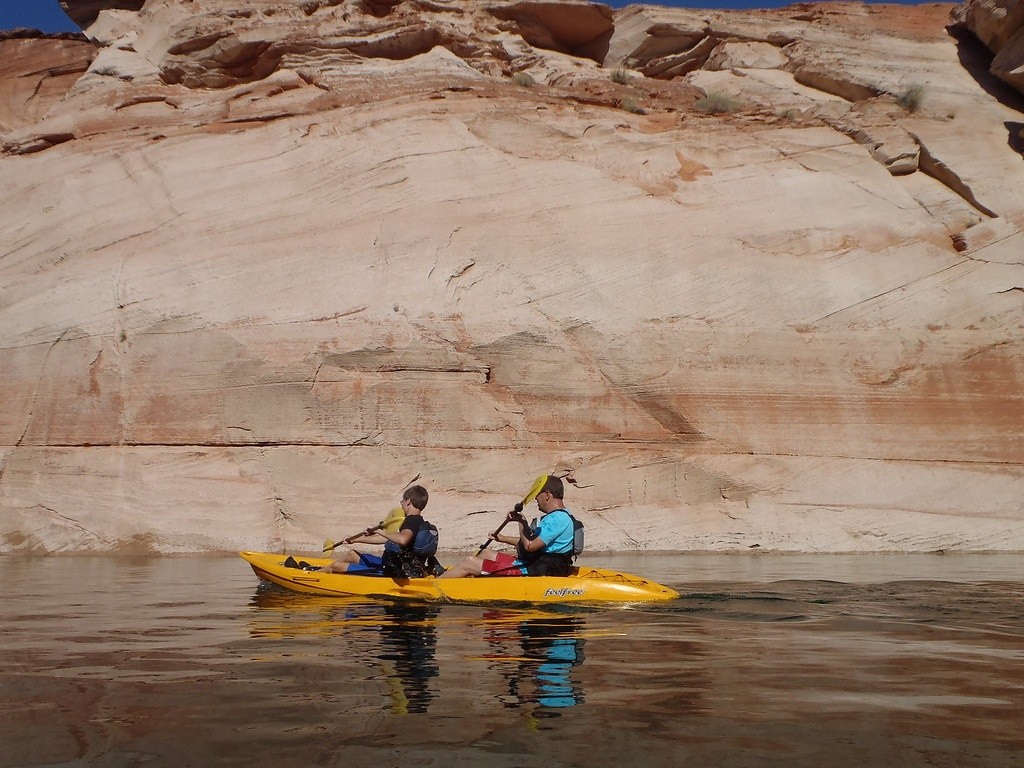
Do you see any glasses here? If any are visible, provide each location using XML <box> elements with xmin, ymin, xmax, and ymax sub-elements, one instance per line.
<box><xmin>400</xmin><ymin>500</ymin><xmax>403</xmax><ymax>505</ymax></box>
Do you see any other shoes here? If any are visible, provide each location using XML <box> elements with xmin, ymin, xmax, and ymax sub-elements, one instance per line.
<box><xmin>299</xmin><ymin>560</ymin><xmax>314</xmax><ymax>571</ymax></box>
<box><xmin>286</xmin><ymin>557</ymin><xmax>300</xmax><ymax>569</ymax></box>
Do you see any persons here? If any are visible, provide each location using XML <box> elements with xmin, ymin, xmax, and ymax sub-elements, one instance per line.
<box><xmin>438</xmin><ymin>475</ymin><xmax>584</xmax><ymax>578</ymax></box>
<box><xmin>285</xmin><ymin>485</ymin><xmax>439</xmax><ymax>573</ymax></box>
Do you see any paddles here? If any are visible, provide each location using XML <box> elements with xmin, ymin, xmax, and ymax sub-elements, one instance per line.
<box><xmin>475</xmin><ymin>474</ymin><xmax>548</xmax><ymax>557</ymax></box>
<box><xmin>322</xmin><ymin>508</ymin><xmax>406</xmax><ymax>559</ymax></box>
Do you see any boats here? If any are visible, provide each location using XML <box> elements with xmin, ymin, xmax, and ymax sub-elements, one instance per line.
<box><xmin>236</xmin><ymin>546</ymin><xmax>680</xmax><ymax>604</ymax></box>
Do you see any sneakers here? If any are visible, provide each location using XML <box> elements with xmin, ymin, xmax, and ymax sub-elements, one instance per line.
<box><xmin>428</xmin><ymin>557</ymin><xmax>445</xmax><ymax>577</ymax></box>
<box><xmin>402</xmin><ymin>563</ymin><xmax>420</xmax><ymax>578</ymax></box>
